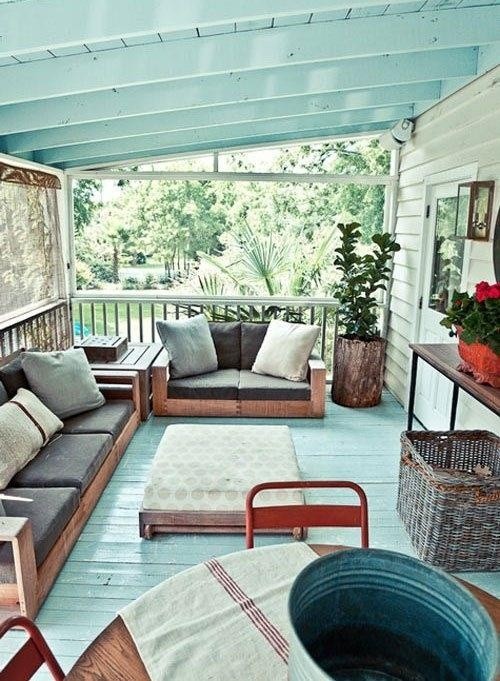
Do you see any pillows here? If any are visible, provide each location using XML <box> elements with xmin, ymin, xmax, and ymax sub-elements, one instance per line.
<box><xmin>241</xmin><ymin>321</ymin><xmax>270</xmax><ymax>369</ymax></box>
<box><xmin>155</xmin><ymin>313</ymin><xmax>219</xmax><ymax>379</ymax></box>
<box><xmin>0</xmin><ymin>346</ymin><xmax>106</xmax><ymax>489</ymax></box>
<box><xmin>208</xmin><ymin>320</ymin><xmax>240</xmax><ymax>369</ymax></box>
<box><xmin>251</xmin><ymin>318</ymin><xmax>322</xmax><ymax>381</ymax></box>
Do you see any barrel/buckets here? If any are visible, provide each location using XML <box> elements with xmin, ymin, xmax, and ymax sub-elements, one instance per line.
<box><xmin>285</xmin><ymin>548</ymin><xmax>500</xmax><ymax>681</ymax></box>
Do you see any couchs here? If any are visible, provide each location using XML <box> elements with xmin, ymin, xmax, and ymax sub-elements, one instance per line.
<box><xmin>151</xmin><ymin>344</ymin><xmax>327</xmax><ymax>420</ymax></box>
<box><xmin>0</xmin><ymin>343</ymin><xmax>142</xmax><ymax>630</ymax></box>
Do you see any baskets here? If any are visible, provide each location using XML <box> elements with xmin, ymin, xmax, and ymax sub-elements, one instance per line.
<box><xmin>397</xmin><ymin>430</ymin><xmax>500</xmax><ymax>573</ymax></box>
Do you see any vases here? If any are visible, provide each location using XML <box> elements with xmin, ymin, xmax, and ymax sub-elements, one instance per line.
<box><xmin>451</xmin><ymin>323</ymin><xmax>499</xmax><ymax>392</ymax></box>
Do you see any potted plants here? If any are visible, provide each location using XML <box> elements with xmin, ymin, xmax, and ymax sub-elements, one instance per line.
<box><xmin>329</xmin><ymin>221</ymin><xmax>402</xmax><ymax>408</ymax></box>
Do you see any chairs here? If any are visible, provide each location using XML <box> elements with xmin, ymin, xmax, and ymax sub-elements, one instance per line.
<box><xmin>244</xmin><ymin>479</ymin><xmax>369</xmax><ymax>551</ymax></box>
<box><xmin>1</xmin><ymin>613</ymin><xmax>66</xmax><ymax>681</ymax></box>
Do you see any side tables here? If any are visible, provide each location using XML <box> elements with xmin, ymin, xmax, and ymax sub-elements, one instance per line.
<box><xmin>64</xmin><ymin>334</ymin><xmax>164</xmax><ymax>422</ymax></box>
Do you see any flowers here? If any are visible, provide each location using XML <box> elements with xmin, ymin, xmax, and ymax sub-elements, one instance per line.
<box><xmin>440</xmin><ymin>281</ymin><xmax>500</xmax><ymax>354</ymax></box>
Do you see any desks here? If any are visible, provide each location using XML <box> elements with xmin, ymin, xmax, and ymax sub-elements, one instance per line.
<box><xmin>62</xmin><ymin>543</ymin><xmax>499</xmax><ymax>680</ymax></box>
<box><xmin>407</xmin><ymin>342</ymin><xmax>499</xmax><ymax>431</ymax></box>
